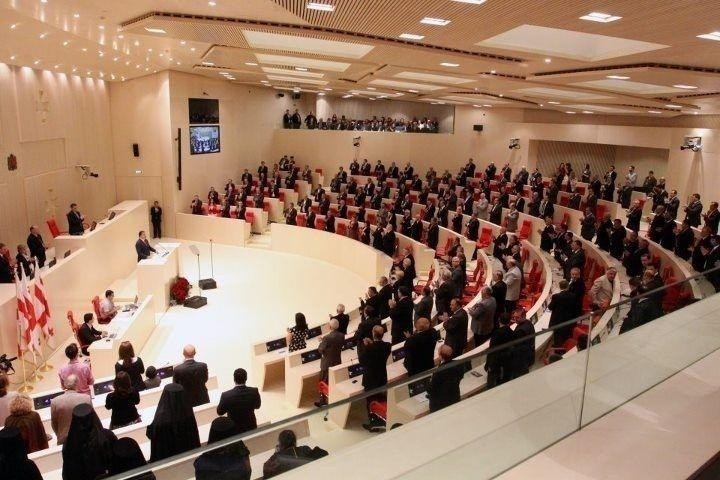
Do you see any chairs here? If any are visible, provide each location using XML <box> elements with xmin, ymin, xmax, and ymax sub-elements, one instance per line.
<box><xmin>67</xmin><ymin>310</ymin><xmax>90</xmax><ymax>358</ymax></box>
<box><xmin>46</xmin><ymin>219</ymin><xmax>69</xmax><ymax>240</ymax></box>
<box><xmin>92</xmin><ymin>295</ymin><xmax>111</xmax><ymax>325</ymax></box>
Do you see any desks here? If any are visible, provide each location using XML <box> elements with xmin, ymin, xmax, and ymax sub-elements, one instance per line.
<box><xmin>0</xmin><ymin>247</ymin><xmax>95</xmax><ymax>392</ymax></box>
<box><xmin>88</xmin><ymin>293</ymin><xmax>157</xmax><ymax>382</ymax></box>
<box><xmin>54</xmin><ymin>200</ymin><xmax>150</xmax><ymax>295</ymax></box>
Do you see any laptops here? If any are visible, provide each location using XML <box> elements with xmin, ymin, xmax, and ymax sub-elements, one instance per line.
<box><xmin>122</xmin><ymin>294</ymin><xmax>138</xmax><ymax>312</ymax></box>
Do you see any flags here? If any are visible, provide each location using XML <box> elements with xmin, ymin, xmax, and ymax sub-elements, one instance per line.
<box><xmin>15</xmin><ymin>273</ymin><xmax>56</xmax><ymax>357</ymax></box>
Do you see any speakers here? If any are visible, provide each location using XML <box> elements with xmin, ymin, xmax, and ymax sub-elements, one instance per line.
<box><xmin>133</xmin><ymin>143</ymin><xmax>139</xmax><ymax>157</ymax></box>
<box><xmin>292</xmin><ymin>93</ymin><xmax>301</xmax><ymax>99</ymax></box>
<box><xmin>473</xmin><ymin>125</ymin><xmax>483</xmax><ymax>131</ymax></box>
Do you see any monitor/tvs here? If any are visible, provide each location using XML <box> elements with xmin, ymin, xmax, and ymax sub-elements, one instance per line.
<box><xmin>591</xmin><ymin>306</ymin><xmax>620</xmax><ymax>346</ymax></box>
<box><xmin>48</xmin><ymin>258</ymin><xmax>56</xmax><ymax>268</ymax></box>
<box><xmin>89</xmin><ymin>220</ymin><xmax>98</xmax><ymax>231</ymax></box>
<box><xmin>189</xmin><ymin>123</ymin><xmax>220</xmax><ymax>155</ymax></box>
<box><xmin>93</xmin><ymin>379</ymin><xmax>115</xmax><ymax>395</ymax></box>
<box><xmin>530</xmin><ymin>287</ymin><xmax>552</xmax><ymax>326</ymax></box>
<box><xmin>266</xmin><ymin>323</ymin><xmax>472</xmax><ymax>398</ymax></box>
<box><xmin>156</xmin><ymin>365</ymin><xmax>173</xmax><ymax>379</ymax></box>
<box><xmin>33</xmin><ymin>391</ymin><xmax>65</xmax><ymax>410</ymax></box>
<box><xmin>108</xmin><ymin>210</ymin><xmax>116</xmax><ymax>220</ymax></box>
<box><xmin>63</xmin><ymin>249</ymin><xmax>71</xmax><ymax>258</ymax></box>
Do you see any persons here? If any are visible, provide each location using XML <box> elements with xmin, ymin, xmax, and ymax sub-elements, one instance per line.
<box><xmin>50</xmin><ymin>374</ymin><xmax>93</xmax><ymax>445</ymax></box>
<box><xmin>105</xmin><ymin>371</ymin><xmax>142</xmax><ymax>430</ymax></box>
<box><xmin>79</xmin><ymin>313</ymin><xmax>108</xmax><ymax>356</ymax></box>
<box><xmin>5</xmin><ymin>394</ymin><xmax>49</xmax><ymax>454</ymax></box>
<box><xmin>151</xmin><ymin>201</ymin><xmax>162</xmax><ymax>239</ymax></box>
<box><xmin>190</xmin><ymin>154</ymin><xmax>311</xmax><ymax>220</ymax></box>
<box><xmin>98</xmin><ymin>290</ymin><xmax>123</xmax><ymax>320</ymax></box>
<box><xmin>143</xmin><ymin>366</ymin><xmax>161</xmax><ymax>390</ymax></box>
<box><xmin>27</xmin><ymin>225</ymin><xmax>48</xmax><ymax>268</ymax></box>
<box><xmin>194</xmin><ymin>136</ymin><xmax>218</xmax><ymax>151</ymax></box>
<box><xmin>193</xmin><ymin>416</ymin><xmax>252</xmax><ymax>480</ymax></box>
<box><xmin>283</xmin><ymin>156</ymin><xmax>720</xmax><ymax>432</ymax></box>
<box><xmin>15</xmin><ymin>244</ymin><xmax>35</xmax><ymax>280</ymax></box>
<box><xmin>114</xmin><ymin>341</ymin><xmax>147</xmax><ymax>391</ymax></box>
<box><xmin>146</xmin><ymin>384</ymin><xmax>201</xmax><ymax>463</ymax></box>
<box><xmin>135</xmin><ymin>231</ymin><xmax>158</xmax><ymax>262</ymax></box>
<box><xmin>263</xmin><ymin>430</ymin><xmax>312</xmax><ymax>480</ymax></box>
<box><xmin>62</xmin><ymin>403</ymin><xmax>118</xmax><ymax>480</ymax></box>
<box><xmin>0</xmin><ymin>426</ymin><xmax>44</xmax><ymax>480</ymax></box>
<box><xmin>173</xmin><ymin>345</ymin><xmax>210</xmax><ymax>406</ymax></box>
<box><xmin>284</xmin><ymin>108</ymin><xmax>439</xmax><ymax>134</ymax></box>
<box><xmin>96</xmin><ymin>437</ymin><xmax>156</xmax><ymax>480</ymax></box>
<box><xmin>0</xmin><ymin>242</ymin><xmax>12</xmax><ymax>283</ymax></box>
<box><xmin>0</xmin><ymin>375</ymin><xmax>21</xmax><ymax>427</ymax></box>
<box><xmin>67</xmin><ymin>203</ymin><xmax>85</xmax><ymax>235</ymax></box>
<box><xmin>59</xmin><ymin>343</ymin><xmax>95</xmax><ymax>399</ymax></box>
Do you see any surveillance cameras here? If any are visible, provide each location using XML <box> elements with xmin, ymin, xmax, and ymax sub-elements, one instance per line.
<box><xmin>354</xmin><ymin>142</ymin><xmax>360</xmax><ymax>147</ymax></box>
<box><xmin>509</xmin><ymin>143</ymin><xmax>517</xmax><ymax>149</ymax></box>
<box><xmin>89</xmin><ymin>171</ymin><xmax>99</xmax><ymax>178</ymax></box>
<box><xmin>680</xmin><ymin>141</ymin><xmax>694</xmax><ymax>151</ymax></box>
